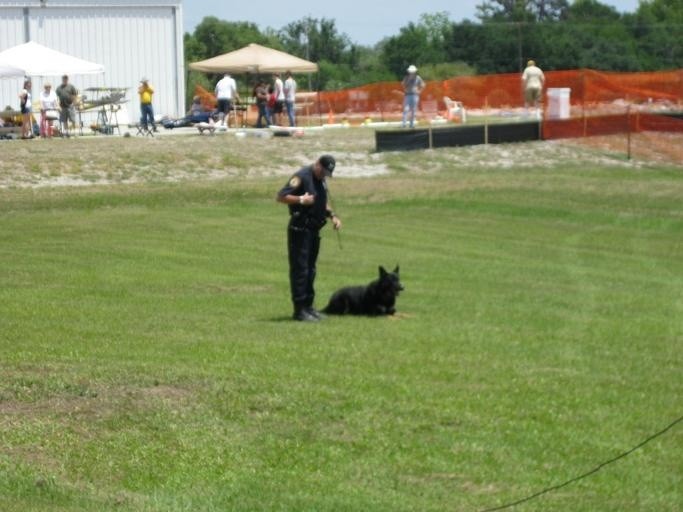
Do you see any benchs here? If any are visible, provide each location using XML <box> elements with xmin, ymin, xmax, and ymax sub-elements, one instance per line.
<box><xmin>196</xmin><ymin>122</ymin><xmax>219</xmax><ymax>135</ymax></box>
<box><xmin>128</xmin><ymin>123</ymin><xmax>159</xmax><ymax>136</ymax></box>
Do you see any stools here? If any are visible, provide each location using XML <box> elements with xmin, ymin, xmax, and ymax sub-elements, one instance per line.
<box><xmin>45</xmin><ymin>116</ymin><xmax>58</xmax><ymax>139</ymax></box>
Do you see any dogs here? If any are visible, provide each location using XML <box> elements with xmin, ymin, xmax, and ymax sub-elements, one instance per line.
<box><xmin>316</xmin><ymin>263</ymin><xmax>405</xmax><ymax>316</ymax></box>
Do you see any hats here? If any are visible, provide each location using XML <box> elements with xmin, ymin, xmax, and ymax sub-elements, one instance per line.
<box><xmin>318</xmin><ymin>155</ymin><xmax>335</xmax><ymax>178</ymax></box>
<box><xmin>406</xmin><ymin>65</ymin><xmax>418</xmax><ymax>71</ymax></box>
<box><xmin>139</xmin><ymin>76</ymin><xmax>149</xmax><ymax>83</ymax></box>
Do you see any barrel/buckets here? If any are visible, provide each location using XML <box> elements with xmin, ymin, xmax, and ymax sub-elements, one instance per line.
<box><xmin>448</xmin><ymin>101</ymin><xmax>465</xmax><ymax>123</ymax></box>
<box><xmin>546</xmin><ymin>87</ymin><xmax>572</xmax><ymax>118</ymax></box>
<box><xmin>422</xmin><ymin>100</ymin><xmax>437</xmax><ymax>113</ymax></box>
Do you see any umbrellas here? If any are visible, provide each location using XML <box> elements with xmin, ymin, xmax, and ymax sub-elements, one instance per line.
<box><xmin>0</xmin><ymin>38</ymin><xmax>114</xmax><ymax>81</ymax></box>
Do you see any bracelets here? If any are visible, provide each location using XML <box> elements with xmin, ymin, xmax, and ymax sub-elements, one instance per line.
<box><xmin>300</xmin><ymin>195</ymin><xmax>304</xmax><ymax>205</ymax></box>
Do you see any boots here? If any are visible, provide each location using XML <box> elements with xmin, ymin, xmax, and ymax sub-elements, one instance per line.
<box><xmin>292</xmin><ymin>301</ymin><xmax>328</xmax><ymax>322</ymax></box>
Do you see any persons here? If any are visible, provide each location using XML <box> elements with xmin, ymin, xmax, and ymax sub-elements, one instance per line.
<box><xmin>20</xmin><ymin>76</ymin><xmax>81</xmax><ymax>138</ymax></box>
<box><xmin>276</xmin><ymin>157</ymin><xmax>340</xmax><ymax>323</ymax></box>
<box><xmin>137</xmin><ymin>70</ymin><xmax>298</xmax><ymax>133</ymax></box>
<box><xmin>400</xmin><ymin>65</ymin><xmax>425</xmax><ymax>129</ymax></box>
<box><xmin>521</xmin><ymin>59</ymin><xmax>544</xmax><ymax>117</ymax></box>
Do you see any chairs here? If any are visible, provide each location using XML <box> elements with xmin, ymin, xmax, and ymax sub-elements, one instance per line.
<box><xmin>443</xmin><ymin>95</ymin><xmax>467</xmax><ymax>123</ymax></box>
